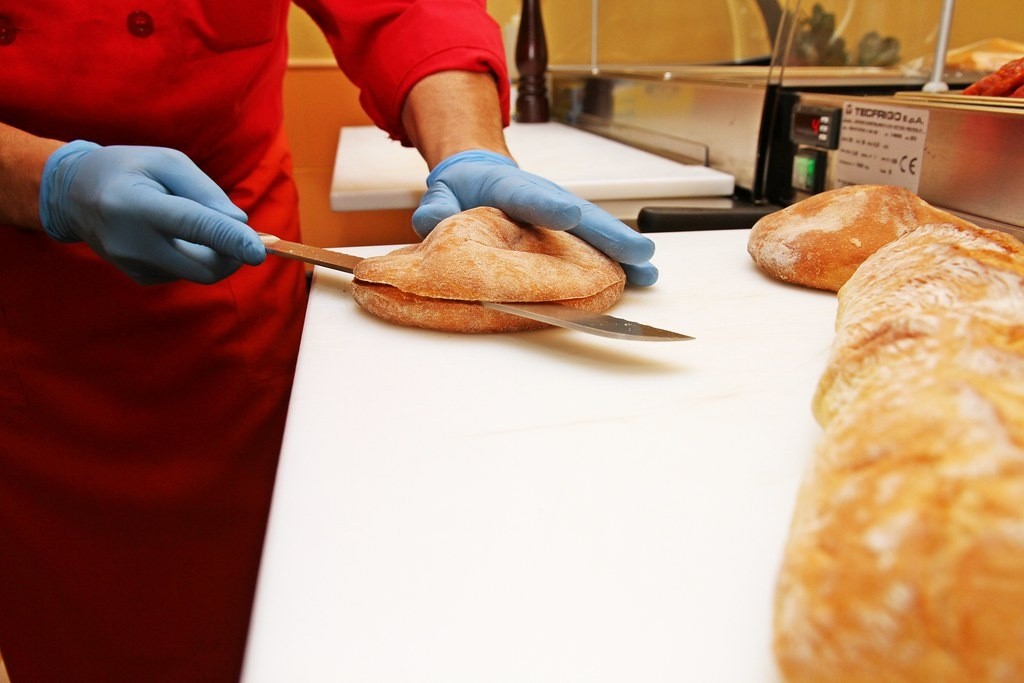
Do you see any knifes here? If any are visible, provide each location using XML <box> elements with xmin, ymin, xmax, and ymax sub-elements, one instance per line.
<box><xmin>257</xmin><ymin>232</ymin><xmax>696</xmax><ymax>343</ymax></box>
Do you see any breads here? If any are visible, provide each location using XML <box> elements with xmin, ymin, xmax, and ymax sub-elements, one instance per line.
<box><xmin>744</xmin><ymin>183</ymin><xmax>1024</xmax><ymax>683</ymax></box>
<box><xmin>350</xmin><ymin>204</ymin><xmax>627</xmax><ymax>334</ymax></box>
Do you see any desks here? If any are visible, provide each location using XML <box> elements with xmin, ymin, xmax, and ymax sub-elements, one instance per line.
<box><xmin>327</xmin><ymin>118</ymin><xmax>740</xmax><ymax>211</ymax></box>
<box><xmin>243</xmin><ymin>227</ymin><xmax>840</xmax><ymax>683</ymax></box>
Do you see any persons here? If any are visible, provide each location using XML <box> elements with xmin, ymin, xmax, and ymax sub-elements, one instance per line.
<box><xmin>0</xmin><ymin>0</ymin><xmax>659</xmax><ymax>683</ymax></box>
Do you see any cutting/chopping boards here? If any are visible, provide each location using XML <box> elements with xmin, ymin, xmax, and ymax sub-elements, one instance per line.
<box><xmin>329</xmin><ymin>121</ymin><xmax>735</xmax><ymax>212</ymax></box>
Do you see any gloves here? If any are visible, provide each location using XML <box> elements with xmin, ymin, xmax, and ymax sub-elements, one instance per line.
<box><xmin>412</xmin><ymin>149</ymin><xmax>660</xmax><ymax>287</ymax></box>
<box><xmin>38</xmin><ymin>141</ymin><xmax>265</xmax><ymax>288</ymax></box>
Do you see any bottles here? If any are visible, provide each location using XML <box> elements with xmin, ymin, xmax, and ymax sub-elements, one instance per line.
<box><xmin>515</xmin><ymin>0</ymin><xmax>550</xmax><ymax>123</ymax></box>
